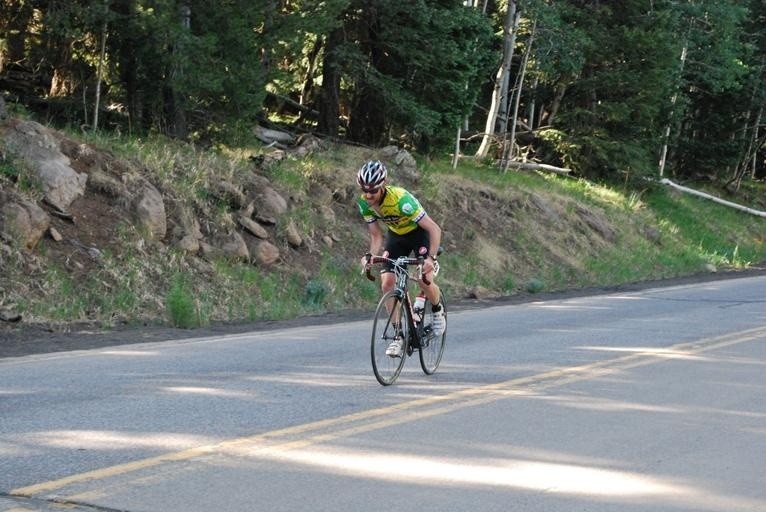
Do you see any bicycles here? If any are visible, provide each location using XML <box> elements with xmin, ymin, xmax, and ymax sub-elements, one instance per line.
<box><xmin>363</xmin><ymin>248</ymin><xmax>452</xmax><ymax>390</ymax></box>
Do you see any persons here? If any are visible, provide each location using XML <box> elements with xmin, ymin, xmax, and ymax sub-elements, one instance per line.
<box><xmin>356</xmin><ymin>159</ymin><xmax>446</xmax><ymax>357</ymax></box>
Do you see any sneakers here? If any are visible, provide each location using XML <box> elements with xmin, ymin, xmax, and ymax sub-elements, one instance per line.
<box><xmin>433</xmin><ymin>303</ymin><xmax>445</xmax><ymax>337</ymax></box>
<box><xmin>386</xmin><ymin>336</ymin><xmax>404</xmax><ymax>355</ymax></box>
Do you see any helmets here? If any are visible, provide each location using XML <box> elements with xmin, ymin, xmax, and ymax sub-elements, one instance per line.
<box><xmin>357</xmin><ymin>160</ymin><xmax>387</xmax><ymax>190</ymax></box>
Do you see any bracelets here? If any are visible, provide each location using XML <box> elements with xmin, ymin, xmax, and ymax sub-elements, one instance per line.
<box><xmin>427</xmin><ymin>253</ymin><xmax>438</xmax><ymax>261</ymax></box>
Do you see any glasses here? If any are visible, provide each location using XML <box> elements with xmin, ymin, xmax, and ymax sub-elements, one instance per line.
<box><xmin>362</xmin><ymin>188</ymin><xmax>378</xmax><ymax>193</ymax></box>
